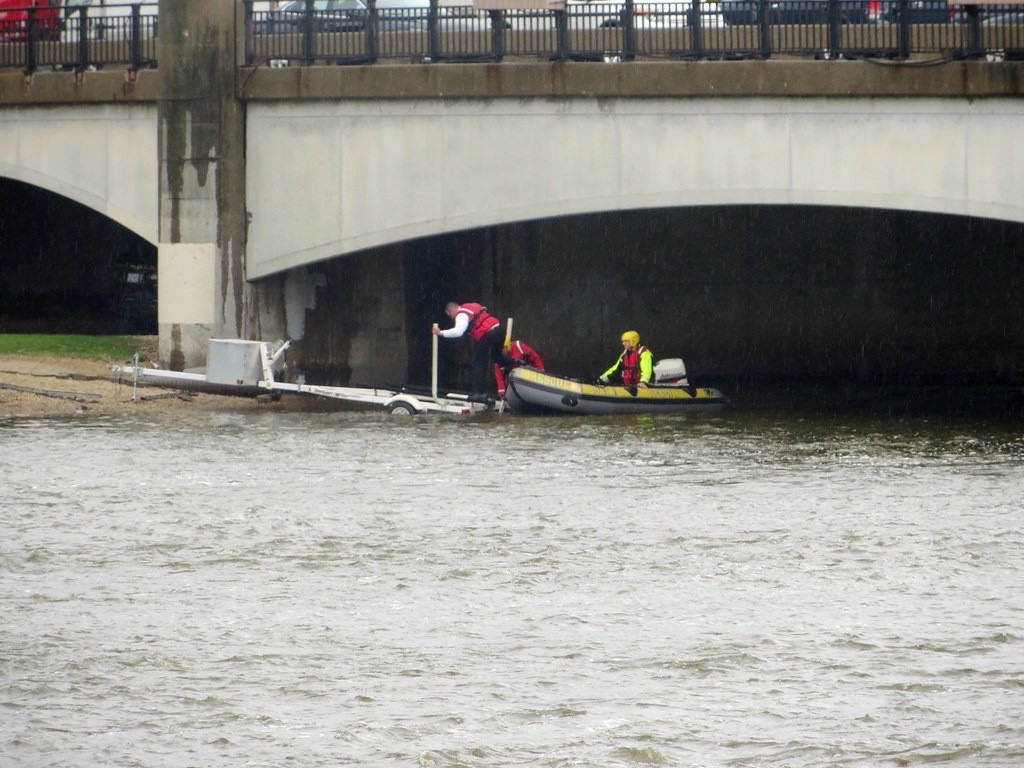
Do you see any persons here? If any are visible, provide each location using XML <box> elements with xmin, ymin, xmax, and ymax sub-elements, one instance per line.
<box><xmin>495</xmin><ymin>339</ymin><xmax>546</xmax><ymax>398</ymax></box>
<box><xmin>593</xmin><ymin>331</ymin><xmax>656</xmax><ymax>390</ymax></box>
<box><xmin>431</xmin><ymin>301</ymin><xmax>502</xmax><ymax>404</ymax></box>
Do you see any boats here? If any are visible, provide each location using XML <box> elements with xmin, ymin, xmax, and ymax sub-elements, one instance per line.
<box><xmin>502</xmin><ymin>363</ymin><xmax>730</xmax><ymax>416</ymax></box>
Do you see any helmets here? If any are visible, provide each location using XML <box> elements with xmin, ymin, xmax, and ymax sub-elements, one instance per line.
<box><xmin>621</xmin><ymin>330</ymin><xmax>640</xmax><ymax>347</ymax></box>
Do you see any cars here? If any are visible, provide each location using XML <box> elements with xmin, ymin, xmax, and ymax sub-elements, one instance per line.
<box><xmin>0</xmin><ymin>0</ymin><xmax>1024</xmax><ymax>41</ymax></box>
